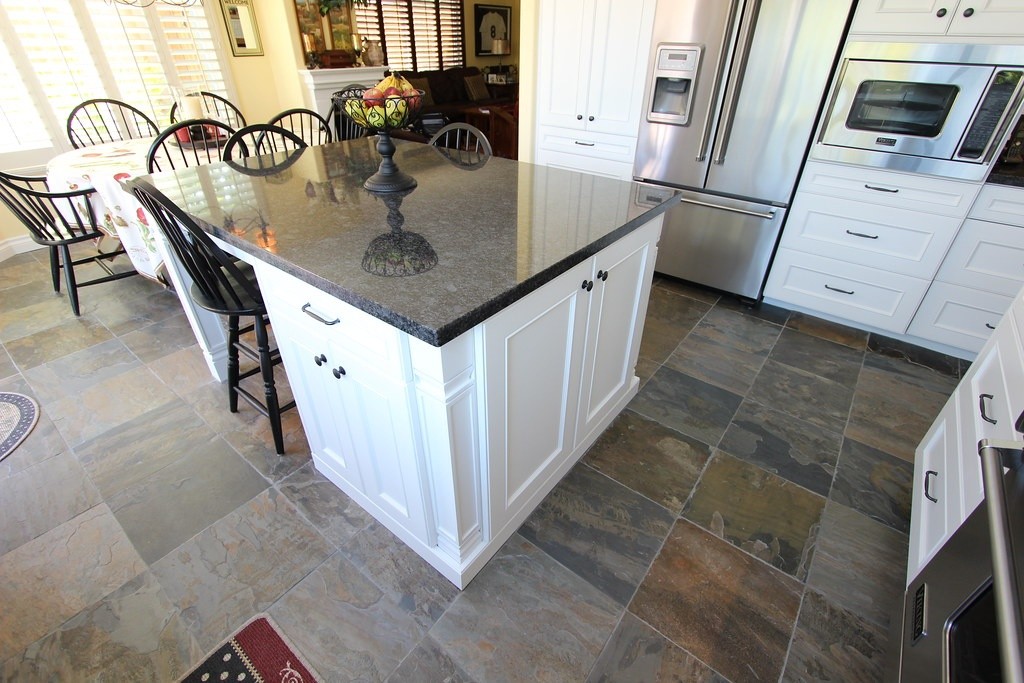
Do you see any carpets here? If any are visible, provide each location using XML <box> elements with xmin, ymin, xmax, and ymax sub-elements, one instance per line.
<box><xmin>0</xmin><ymin>392</ymin><xmax>40</xmax><ymax>463</ymax></box>
<box><xmin>175</xmin><ymin>613</ymin><xmax>325</xmax><ymax>682</ymax></box>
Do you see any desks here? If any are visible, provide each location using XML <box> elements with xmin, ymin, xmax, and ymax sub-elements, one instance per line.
<box><xmin>47</xmin><ymin>125</ymin><xmax>328</xmax><ymax>287</ymax></box>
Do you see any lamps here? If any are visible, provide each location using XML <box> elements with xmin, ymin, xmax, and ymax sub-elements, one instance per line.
<box><xmin>492</xmin><ymin>38</ymin><xmax>510</xmax><ymax>72</ymax></box>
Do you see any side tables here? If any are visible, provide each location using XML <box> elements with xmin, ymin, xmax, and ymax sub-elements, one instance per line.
<box><xmin>485</xmin><ymin>80</ymin><xmax>517</xmax><ymax>102</ymax></box>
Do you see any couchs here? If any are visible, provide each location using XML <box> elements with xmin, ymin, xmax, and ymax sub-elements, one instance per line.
<box><xmin>384</xmin><ymin>66</ymin><xmax>510</xmax><ymax>127</ymax></box>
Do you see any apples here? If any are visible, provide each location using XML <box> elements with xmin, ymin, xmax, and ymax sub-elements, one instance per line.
<box><xmin>402</xmin><ymin>90</ymin><xmax>421</xmax><ymax>111</ymax></box>
<box><xmin>362</xmin><ymin>88</ymin><xmax>384</xmax><ymax>107</ymax></box>
<box><xmin>382</xmin><ymin>86</ymin><xmax>401</xmax><ymax>99</ymax></box>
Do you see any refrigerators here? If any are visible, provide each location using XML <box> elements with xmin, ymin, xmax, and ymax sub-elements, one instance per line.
<box><xmin>633</xmin><ymin>1</ymin><xmax>853</xmax><ymax>300</ymax></box>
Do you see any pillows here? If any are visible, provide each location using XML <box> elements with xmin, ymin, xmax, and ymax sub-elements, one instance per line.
<box><xmin>406</xmin><ymin>77</ymin><xmax>435</xmax><ymax>107</ymax></box>
<box><xmin>463</xmin><ymin>75</ymin><xmax>490</xmax><ymax>103</ymax></box>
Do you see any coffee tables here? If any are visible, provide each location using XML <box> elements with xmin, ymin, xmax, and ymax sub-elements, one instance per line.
<box><xmin>456</xmin><ymin>103</ymin><xmax>514</xmax><ymax>148</ymax></box>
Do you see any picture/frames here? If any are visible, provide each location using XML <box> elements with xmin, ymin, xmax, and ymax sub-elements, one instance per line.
<box><xmin>473</xmin><ymin>3</ymin><xmax>512</xmax><ymax>57</ymax></box>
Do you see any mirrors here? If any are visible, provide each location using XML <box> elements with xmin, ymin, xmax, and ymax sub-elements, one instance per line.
<box><xmin>219</xmin><ymin>0</ymin><xmax>264</xmax><ymax>57</ymax></box>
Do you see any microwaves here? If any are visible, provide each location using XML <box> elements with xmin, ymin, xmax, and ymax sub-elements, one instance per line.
<box><xmin>809</xmin><ymin>44</ymin><xmax>1024</xmax><ymax>182</ymax></box>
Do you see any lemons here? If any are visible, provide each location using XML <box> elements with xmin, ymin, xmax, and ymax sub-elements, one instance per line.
<box><xmin>345</xmin><ymin>95</ymin><xmax>407</xmax><ymax>127</ymax></box>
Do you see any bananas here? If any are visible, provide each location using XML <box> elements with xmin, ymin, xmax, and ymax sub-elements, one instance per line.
<box><xmin>374</xmin><ymin>70</ymin><xmax>413</xmax><ymax>92</ymax></box>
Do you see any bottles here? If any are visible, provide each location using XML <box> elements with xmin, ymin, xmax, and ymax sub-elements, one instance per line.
<box><xmin>360</xmin><ymin>40</ymin><xmax>384</xmax><ymax>67</ymax></box>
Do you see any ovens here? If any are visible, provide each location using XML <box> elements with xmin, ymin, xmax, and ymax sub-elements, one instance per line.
<box><xmin>899</xmin><ymin>439</ymin><xmax>1024</xmax><ymax>683</ymax></box>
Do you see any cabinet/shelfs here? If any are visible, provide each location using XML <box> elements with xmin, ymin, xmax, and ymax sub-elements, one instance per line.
<box><xmin>141</xmin><ymin>135</ymin><xmax>665</xmax><ymax>549</ymax></box>
<box><xmin>844</xmin><ymin>1</ymin><xmax>1024</xmax><ymax>47</ymax></box>
<box><xmin>534</xmin><ymin>2</ymin><xmax>660</xmax><ymax>182</ymax></box>
<box><xmin>763</xmin><ymin>160</ymin><xmax>981</xmax><ymax>343</ymax></box>
<box><xmin>905</xmin><ymin>287</ymin><xmax>1024</xmax><ymax>587</ymax></box>
<box><xmin>904</xmin><ymin>184</ymin><xmax>1024</xmax><ymax>359</ymax></box>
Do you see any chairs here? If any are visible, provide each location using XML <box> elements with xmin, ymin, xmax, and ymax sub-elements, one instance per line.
<box><xmin>0</xmin><ymin>171</ymin><xmax>170</xmax><ymax>317</ymax></box>
<box><xmin>67</xmin><ymin>84</ymin><xmax>375</xmax><ymax>174</ymax></box>
<box><xmin>129</xmin><ymin>176</ymin><xmax>299</xmax><ymax>456</ymax></box>
<box><xmin>428</xmin><ymin>122</ymin><xmax>493</xmax><ymax>158</ymax></box>
<box><xmin>488</xmin><ymin>88</ymin><xmax>518</xmax><ymax>159</ymax></box>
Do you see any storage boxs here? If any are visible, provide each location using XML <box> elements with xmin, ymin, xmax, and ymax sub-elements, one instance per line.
<box><xmin>321</xmin><ymin>50</ymin><xmax>354</xmax><ymax>69</ymax></box>
